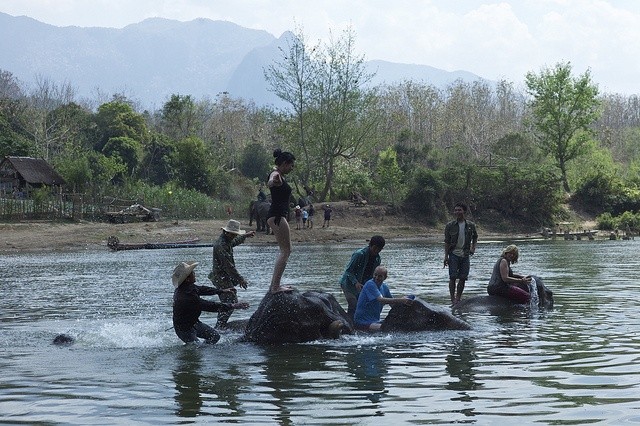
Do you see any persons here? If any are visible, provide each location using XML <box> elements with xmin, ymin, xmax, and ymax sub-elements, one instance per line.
<box><xmin>295</xmin><ymin>205</ymin><xmax>302</xmax><ymax>230</ymax></box>
<box><xmin>304</xmin><ymin>186</ymin><xmax>311</xmax><ymax>195</ymax></box>
<box><xmin>352</xmin><ymin>265</ymin><xmax>413</xmax><ymax>332</ymax></box>
<box><xmin>265</xmin><ymin>147</ymin><xmax>297</xmax><ymax>293</ymax></box>
<box><xmin>169</xmin><ymin>261</ymin><xmax>250</xmax><ymax>347</ymax></box>
<box><xmin>257</xmin><ymin>189</ymin><xmax>265</xmax><ymax>202</ymax></box>
<box><xmin>312</xmin><ymin>184</ymin><xmax>318</xmax><ymax>197</ymax></box>
<box><xmin>307</xmin><ymin>204</ymin><xmax>315</xmax><ymax>229</ymax></box>
<box><xmin>443</xmin><ymin>203</ymin><xmax>479</xmax><ymax>308</ymax></box>
<box><xmin>339</xmin><ymin>235</ymin><xmax>386</xmax><ymax>320</ymax></box>
<box><xmin>298</xmin><ymin>197</ymin><xmax>303</xmax><ymax>205</ymax></box>
<box><xmin>226</xmin><ymin>206</ymin><xmax>232</xmax><ymax>219</ymax></box>
<box><xmin>321</xmin><ymin>203</ymin><xmax>333</xmax><ymax>228</ymax></box>
<box><xmin>301</xmin><ymin>207</ymin><xmax>308</xmax><ymax>229</ymax></box>
<box><xmin>486</xmin><ymin>243</ymin><xmax>532</xmax><ymax>302</ymax></box>
<box><xmin>207</xmin><ymin>218</ymin><xmax>257</xmax><ymax>335</ymax></box>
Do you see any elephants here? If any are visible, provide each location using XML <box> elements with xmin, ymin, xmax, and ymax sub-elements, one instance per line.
<box><xmin>452</xmin><ymin>274</ymin><xmax>554</xmax><ymax>317</ymax></box>
<box><xmin>381</xmin><ymin>295</ymin><xmax>475</xmax><ymax>333</ymax></box>
<box><xmin>246</xmin><ymin>287</ymin><xmax>355</xmax><ymax>346</ymax></box>
<box><xmin>249</xmin><ymin>199</ymin><xmax>275</xmax><ymax>235</ymax></box>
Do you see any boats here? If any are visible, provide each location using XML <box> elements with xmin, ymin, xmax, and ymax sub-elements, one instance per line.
<box><xmin>541</xmin><ymin>220</ymin><xmax>599</xmax><ymax>237</ymax></box>
<box><xmin>143</xmin><ymin>239</ymin><xmax>219</xmax><ymax>249</ymax></box>
<box><xmin>104</xmin><ymin>237</ymin><xmax>202</xmax><ymax>250</ymax></box>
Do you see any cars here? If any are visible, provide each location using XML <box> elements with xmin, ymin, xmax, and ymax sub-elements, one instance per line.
<box><xmin>105</xmin><ymin>202</ymin><xmax>156</xmax><ymax>223</ymax></box>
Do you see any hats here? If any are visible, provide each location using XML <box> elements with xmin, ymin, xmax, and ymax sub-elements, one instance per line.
<box><xmin>296</xmin><ymin>205</ymin><xmax>300</xmax><ymax>209</ymax></box>
<box><xmin>220</xmin><ymin>219</ymin><xmax>246</xmax><ymax>235</ymax></box>
<box><xmin>172</xmin><ymin>262</ymin><xmax>199</xmax><ymax>288</ymax></box>
